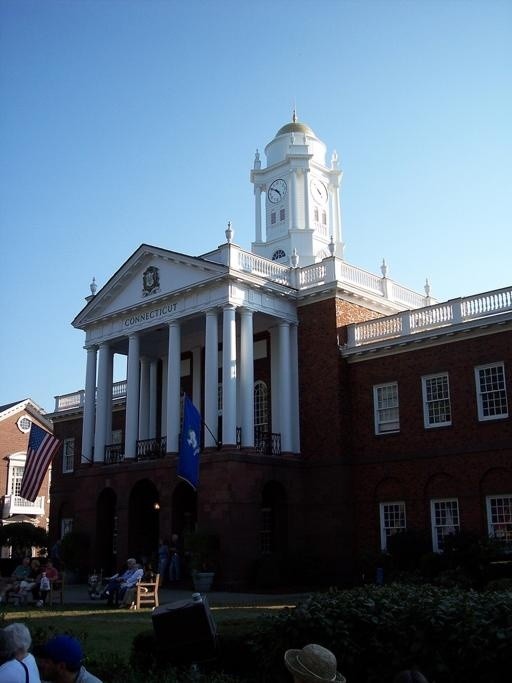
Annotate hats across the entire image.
[33,634,82,665]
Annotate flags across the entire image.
[177,395,203,491]
[18,421,63,501]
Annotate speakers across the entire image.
[150,595,217,664]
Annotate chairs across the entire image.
[134,574,162,610]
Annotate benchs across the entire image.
[3,571,66,606]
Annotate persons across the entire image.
[0,556,156,611]
[157,534,180,603]
[40,635,103,682]
[1,622,41,683]
[51,540,64,560]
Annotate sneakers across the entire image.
[0,595,45,609]
